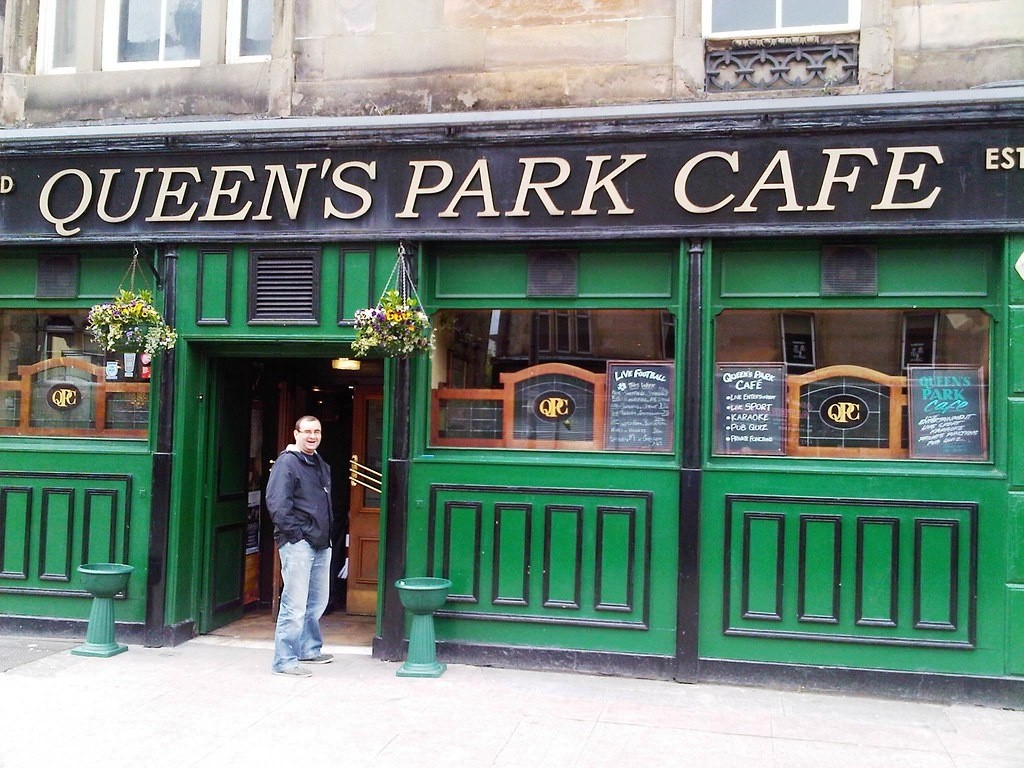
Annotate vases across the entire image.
[393,577,452,679]
[101,323,149,352]
[72,564,134,657]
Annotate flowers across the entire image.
[351,291,436,357]
[86,286,177,354]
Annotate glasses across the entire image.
[298,430,321,437]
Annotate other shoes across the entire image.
[297,654,333,664]
[271,665,312,678]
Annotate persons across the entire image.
[265,416,333,677]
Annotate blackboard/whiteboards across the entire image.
[906,363,986,459]
[603,357,673,453]
[713,359,787,464]
